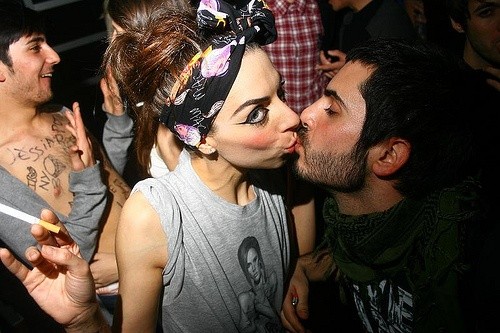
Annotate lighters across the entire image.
[292,292,299,307]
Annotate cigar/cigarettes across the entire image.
[0,203,60,234]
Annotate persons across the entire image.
[107,0,194,190]
[0,6,301,333]
[260,0,329,258]
[397,0,500,192]
[315,0,417,78]
[280,42,500,333]
[0,18,134,327]
